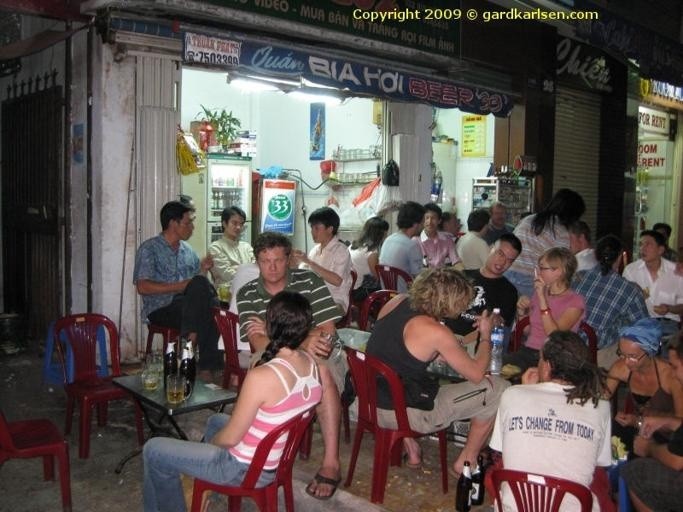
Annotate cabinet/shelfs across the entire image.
[471,177,532,227]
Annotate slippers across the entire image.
[305,472,341,500]
[403,451,422,468]
[447,462,463,479]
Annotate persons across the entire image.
[133,188,682,512]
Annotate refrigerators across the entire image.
[180,151,252,264]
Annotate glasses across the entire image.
[616,349,645,363]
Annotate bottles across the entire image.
[421,253,429,268]
[139,337,196,404]
[210,169,244,233]
[454,454,485,512]
[486,308,504,376]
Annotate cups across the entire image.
[215,283,232,302]
[315,330,342,362]
[334,149,370,183]
